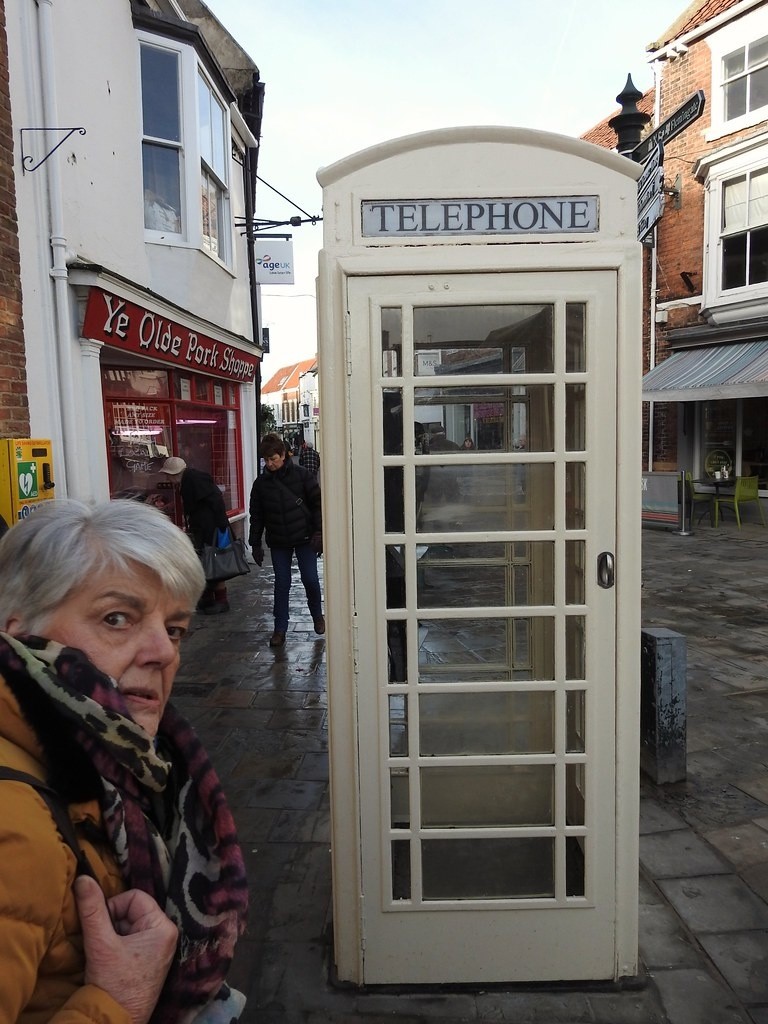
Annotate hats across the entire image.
[159,457,187,474]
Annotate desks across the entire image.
[690,478,742,529]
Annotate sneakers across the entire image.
[312,614,326,635]
[199,589,230,613]
[269,629,287,647]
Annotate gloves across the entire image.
[310,532,323,558]
[250,545,266,567]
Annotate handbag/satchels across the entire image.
[200,526,251,581]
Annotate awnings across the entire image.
[642,338,768,402]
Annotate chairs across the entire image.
[677,472,714,529]
[713,475,767,533]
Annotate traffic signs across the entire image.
[632,90,709,241]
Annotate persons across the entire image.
[248,433,324,646]
[279,406,538,529]
[161,457,234,614]
[1,497,248,1024]
